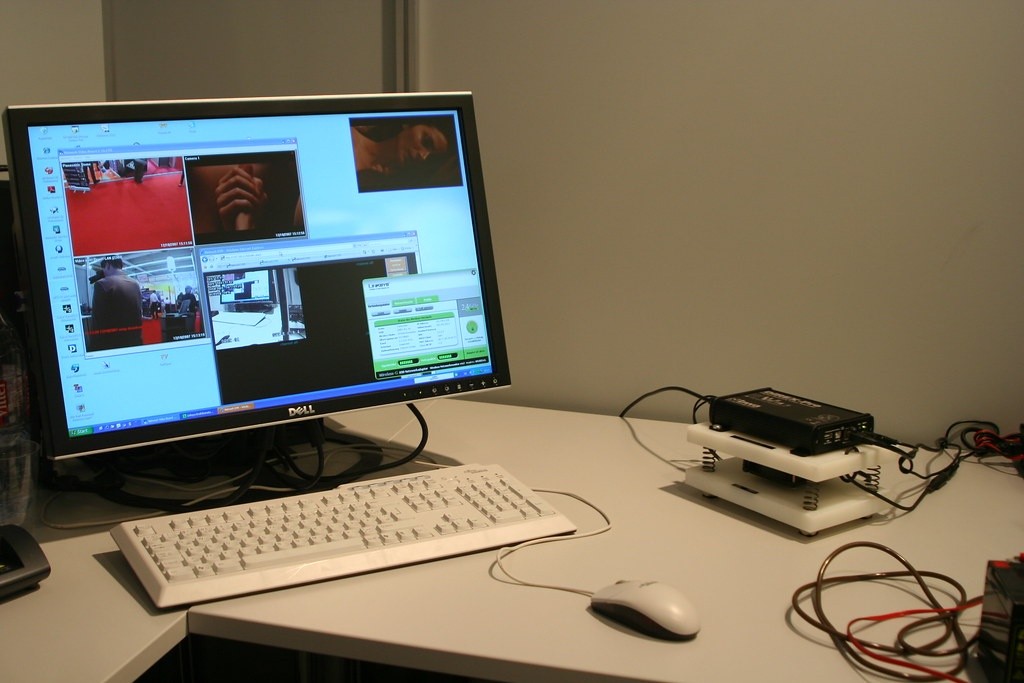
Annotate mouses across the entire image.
[590,580,702,640]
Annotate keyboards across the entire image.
[111,464,578,609]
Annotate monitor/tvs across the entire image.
[1,89,511,509]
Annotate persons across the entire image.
[350,118,453,195]
[187,164,271,234]
[145,285,198,320]
[92,255,143,352]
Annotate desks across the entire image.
[0,398,437,683]
[188,397,1023,683]
